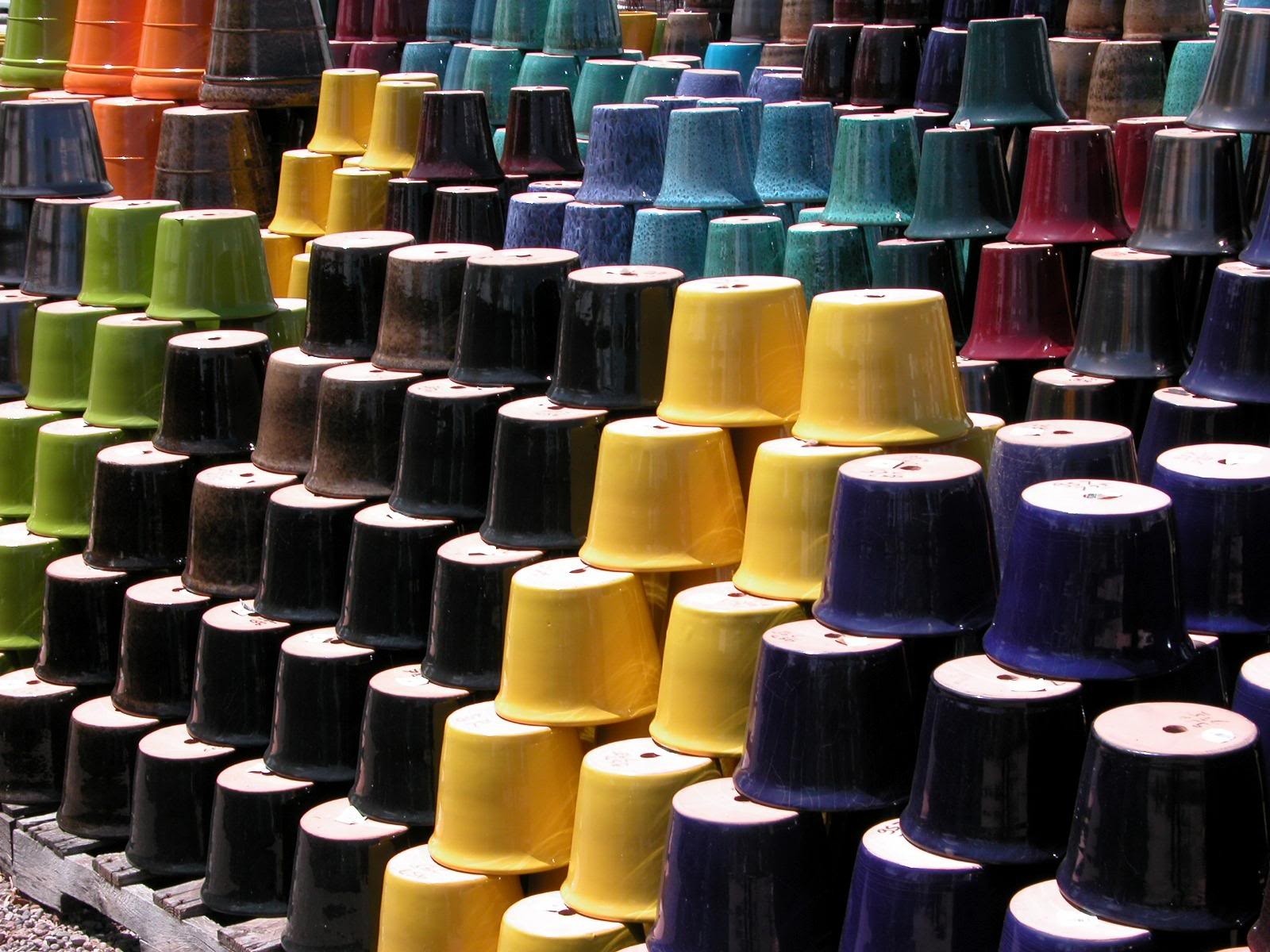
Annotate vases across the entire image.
[0,0,1270,952]
